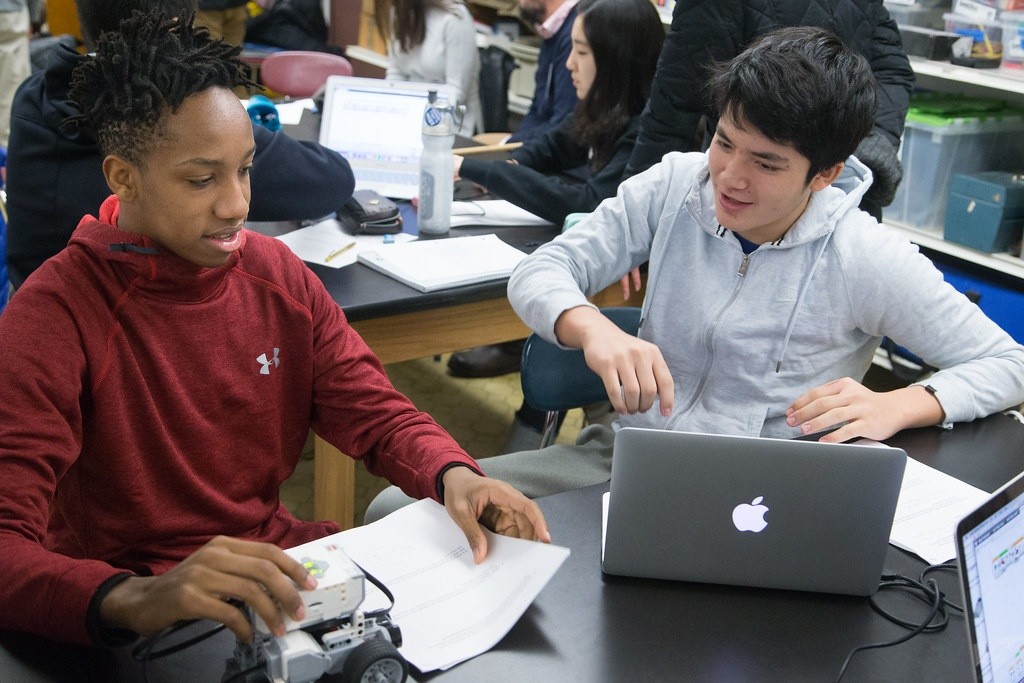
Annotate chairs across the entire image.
[520,307,642,450]
[260,51,353,104]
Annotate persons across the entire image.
[5,0,483,294]
[365,27,1024,526]
[446,0,917,377]
[0,6,551,650]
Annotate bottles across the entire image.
[418,101,455,234]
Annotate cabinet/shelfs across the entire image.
[873,56,1024,379]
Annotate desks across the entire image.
[244,103,648,539]
[0,381,1024,683]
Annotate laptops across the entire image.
[953,472,1024,683]
[319,76,457,201]
[601,426,908,597]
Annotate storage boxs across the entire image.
[882,0,1024,68]
[882,88,1024,236]
[944,170,1024,255]
